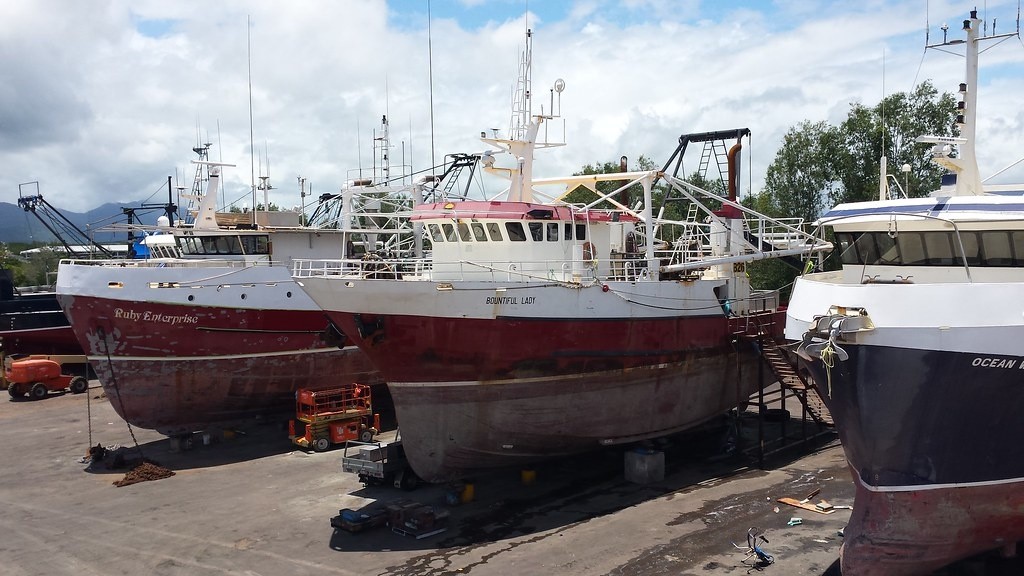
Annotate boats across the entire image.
[54,0,668,440]
[289,0,834,485]
[781,1,1024,576]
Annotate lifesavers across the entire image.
[580,242,595,261]
